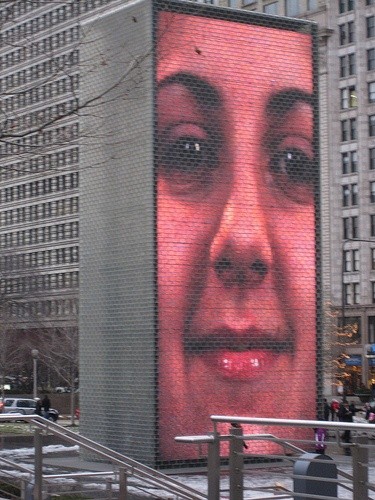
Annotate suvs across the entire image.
[0,397,59,423]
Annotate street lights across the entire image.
[31,348,38,400]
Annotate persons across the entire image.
[154,11,317,459]
[42,395,51,418]
[316,428,326,453]
[34,398,42,415]
[323,397,375,442]
